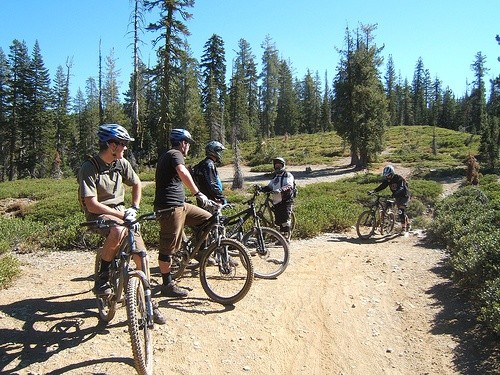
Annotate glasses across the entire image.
[112,141,128,147]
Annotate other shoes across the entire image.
[400,228,405,236]
[407,223,411,231]
[230,258,239,267]
[275,240,290,246]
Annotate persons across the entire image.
[154,129,215,296]
[257,158,294,244]
[80,124,166,324]
[368,167,411,235]
[191,142,238,266]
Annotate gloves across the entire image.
[194,192,208,208]
[127,216,141,233]
[124,206,138,219]
[274,189,281,195]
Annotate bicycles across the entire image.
[250,185,296,241]
[357,193,396,239]
[199,204,289,278]
[80,207,174,375]
[171,223,254,303]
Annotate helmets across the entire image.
[382,167,394,180]
[98,123,135,144]
[170,128,195,144]
[205,141,227,164]
[272,158,286,174]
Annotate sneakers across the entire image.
[161,283,188,296]
[138,302,165,324]
[95,279,112,295]
[198,252,216,266]
